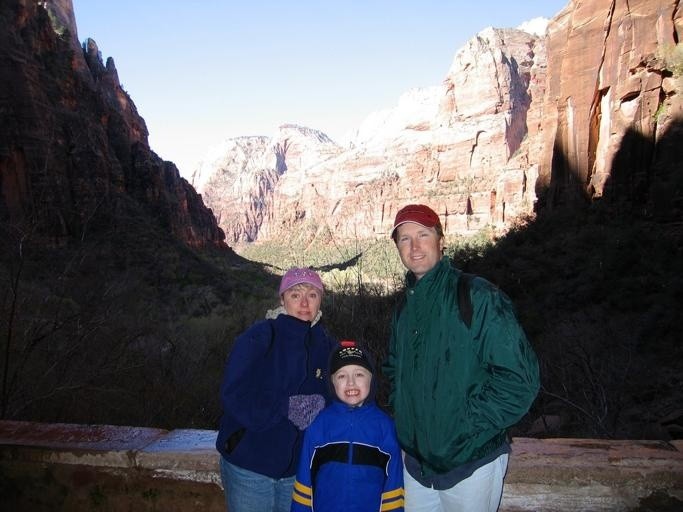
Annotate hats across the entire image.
[330,346,372,375]
[279,267,323,296]
[390,204,439,239]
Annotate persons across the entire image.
[215,266,336,511]
[288,342,402,511]
[380,204,543,512]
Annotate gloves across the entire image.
[288,394,325,432]
